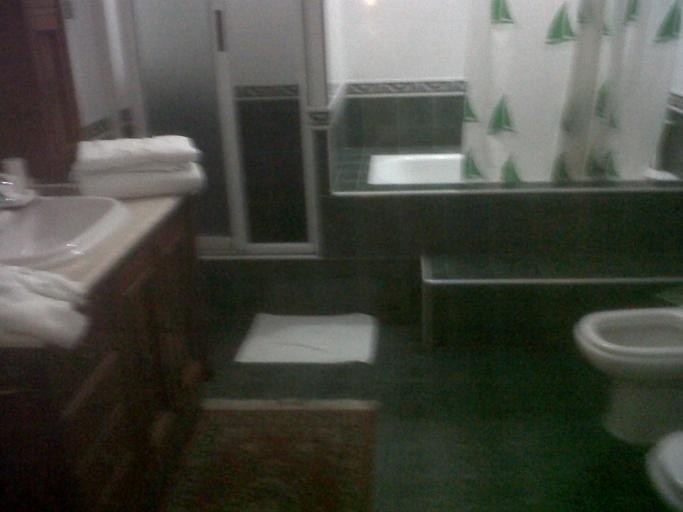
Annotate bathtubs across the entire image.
[363,152,683,188]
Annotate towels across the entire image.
[0,266,89,349]
[77,163,207,199]
[65,135,204,182]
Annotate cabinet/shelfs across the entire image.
[0,198,212,512]
[0,0,88,183]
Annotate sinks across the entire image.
[0,192,122,264]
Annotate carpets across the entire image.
[157,398,380,512]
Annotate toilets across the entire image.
[646,431,683,511]
[573,306,683,441]
